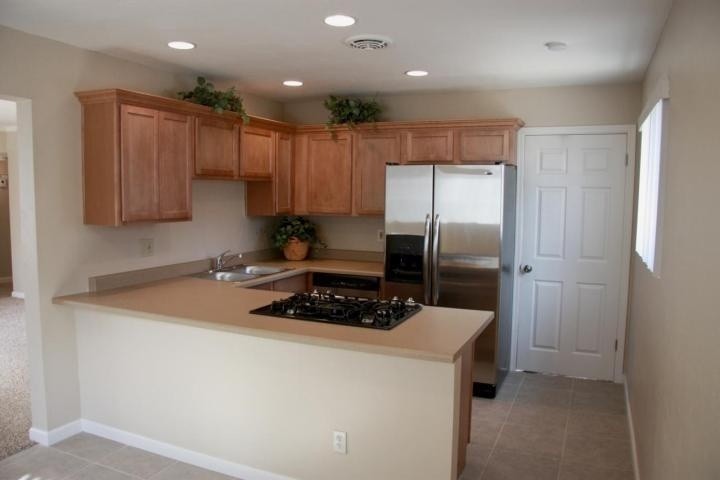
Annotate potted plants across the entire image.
[267,215,328,261]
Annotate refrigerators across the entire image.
[379,158,519,401]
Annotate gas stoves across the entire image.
[246,290,426,330]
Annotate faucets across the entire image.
[214,249,242,271]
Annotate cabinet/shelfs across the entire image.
[406,127,510,163]
[195,115,273,178]
[83,103,193,227]
[247,132,292,218]
[291,126,403,216]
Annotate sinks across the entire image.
[207,271,255,282]
[244,265,285,277]
